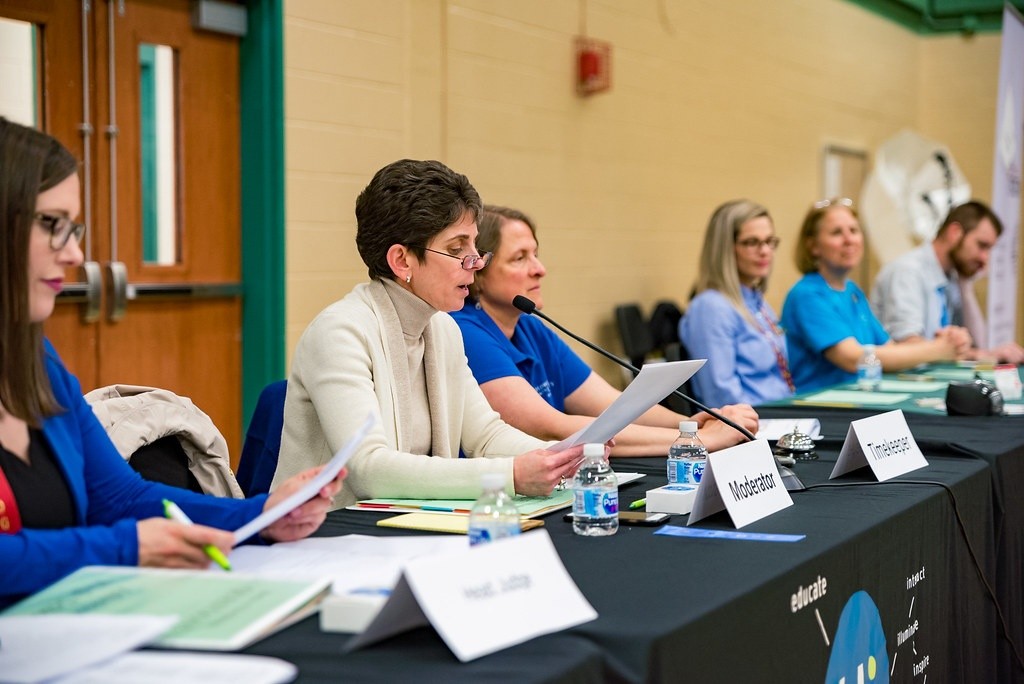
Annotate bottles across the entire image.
[469,472,520,547]
[573,443,619,536]
[857,344,882,391]
[667,422,708,484]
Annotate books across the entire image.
[1,565,336,652]
[377,508,543,535]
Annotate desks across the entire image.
[4,357,1024,684]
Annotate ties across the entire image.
[939,286,948,326]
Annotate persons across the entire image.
[780,195,972,394]
[0,116,349,600]
[867,200,1024,365]
[677,196,796,412]
[448,204,759,458]
[270,157,617,513]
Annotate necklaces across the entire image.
[755,305,795,393]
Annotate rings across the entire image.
[562,475,566,479]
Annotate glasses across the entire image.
[33,211,85,250]
[735,237,780,251]
[815,198,856,214]
[424,245,491,270]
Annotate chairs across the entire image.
[235,380,286,499]
[83,385,245,504]
[617,302,698,418]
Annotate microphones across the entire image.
[512,295,808,488]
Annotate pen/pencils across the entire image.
[162,498,232,571]
[629,498,647,509]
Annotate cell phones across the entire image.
[563,512,671,527]
